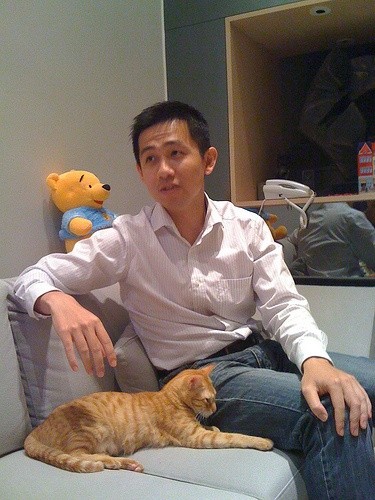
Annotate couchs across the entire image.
[0,276,375,500]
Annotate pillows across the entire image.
[112,320,161,393]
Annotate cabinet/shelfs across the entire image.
[225,0,375,358]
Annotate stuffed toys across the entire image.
[246,207,287,241]
[46,169,120,254]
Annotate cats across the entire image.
[23,361,274,473]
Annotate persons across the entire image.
[12,100,375,500]
[289,201,375,278]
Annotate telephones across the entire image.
[263,179,316,199]
